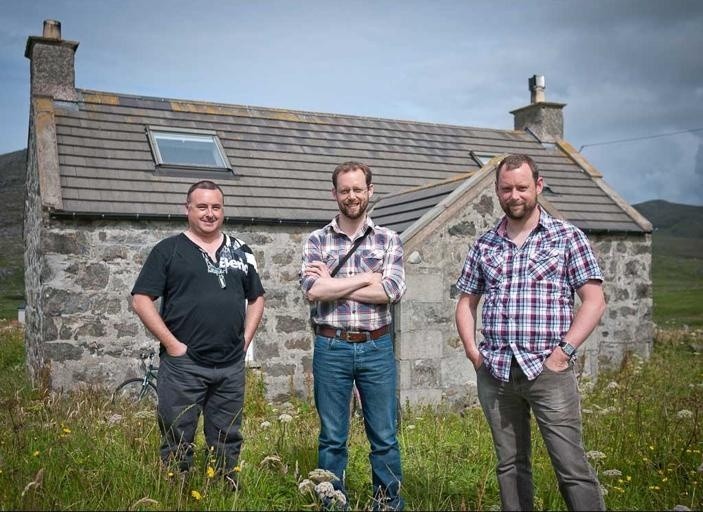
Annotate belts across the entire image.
[314,324,391,343]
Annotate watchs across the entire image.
[558,341,577,357]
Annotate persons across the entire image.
[456,153,607,512]
[132,181,266,490]
[303,161,405,512]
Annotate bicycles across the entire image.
[111,344,263,420]
